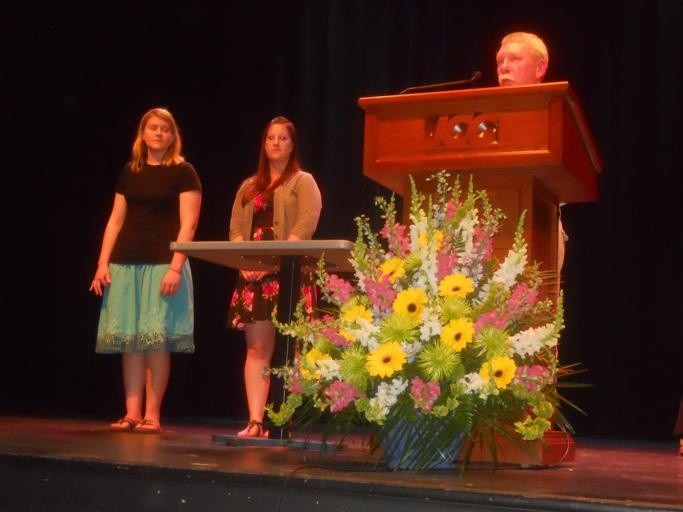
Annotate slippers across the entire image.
[111,418,160,433]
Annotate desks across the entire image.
[166,234,369,456]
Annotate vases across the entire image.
[369,402,481,472]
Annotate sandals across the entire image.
[247,421,263,437]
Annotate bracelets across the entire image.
[168,267,180,274]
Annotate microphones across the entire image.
[399,71,483,94]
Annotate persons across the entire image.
[225,113,322,438]
[88,107,202,432]
[496,31,566,293]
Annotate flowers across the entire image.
[260,168,593,474]
